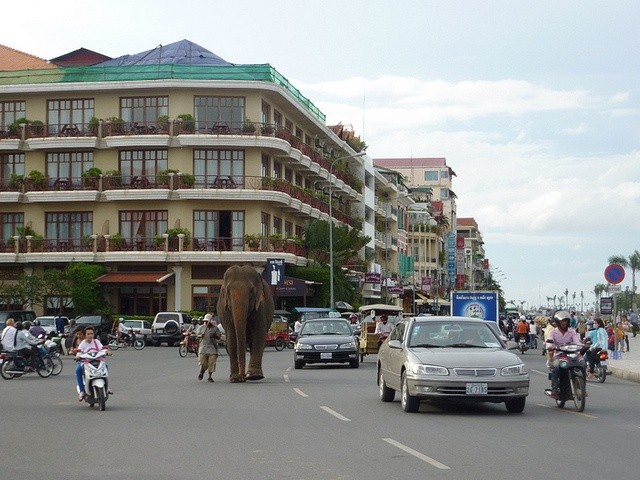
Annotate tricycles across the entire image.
[360,317,368,363]
[261,316,289,352]
[176,319,206,357]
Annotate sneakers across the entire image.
[208,378,214,382]
[199,374,203,380]
[551,389,560,399]
[78,392,85,401]
[107,388,113,394]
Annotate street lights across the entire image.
[329,151,366,309]
[385,195,409,302]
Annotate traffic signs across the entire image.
[602,260,627,284]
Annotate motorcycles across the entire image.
[107,326,145,350]
[518,333,530,355]
[587,348,617,388]
[507,329,513,340]
[72,329,112,410]
[376,332,390,351]
[0,343,63,380]
[542,337,592,412]
[45,343,63,375]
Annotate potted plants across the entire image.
[149,236,165,250]
[27,170,47,191]
[179,113,194,135]
[279,125,367,195]
[105,170,122,188]
[242,119,255,134]
[6,236,15,252]
[156,116,169,134]
[157,170,169,189]
[113,233,125,250]
[275,178,351,225]
[81,234,92,251]
[29,120,45,138]
[7,116,23,138]
[245,233,263,251]
[262,176,272,190]
[82,168,103,190]
[263,125,274,136]
[269,233,284,252]
[286,236,308,256]
[180,174,196,189]
[10,175,20,190]
[165,228,191,249]
[107,116,125,136]
[85,116,100,137]
[16,226,42,253]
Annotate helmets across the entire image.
[349,314,357,319]
[585,320,594,325]
[554,311,571,321]
[22,321,31,328]
[33,319,40,325]
[520,315,526,321]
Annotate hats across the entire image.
[119,318,124,322]
[192,318,200,321]
[379,313,388,318]
[14,321,22,329]
[201,313,215,324]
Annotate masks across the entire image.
[593,324,600,328]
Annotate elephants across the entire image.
[215,264,276,383]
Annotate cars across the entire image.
[2,310,36,328]
[35,316,69,337]
[291,317,361,370]
[149,312,185,346]
[82,342,88,345]
[117,319,150,344]
[376,316,530,414]
[69,315,109,337]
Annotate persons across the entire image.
[294,317,303,334]
[582,318,609,373]
[545,309,590,399]
[69,326,114,402]
[529,321,537,349]
[197,314,221,383]
[542,318,555,367]
[117,317,133,348]
[622,315,632,352]
[499,315,515,334]
[605,320,612,329]
[0,317,47,370]
[515,315,530,344]
[570,311,577,329]
[349,313,363,341]
[375,315,395,352]
[61,318,77,338]
[186,317,200,346]
[615,322,624,352]
[629,310,638,337]
